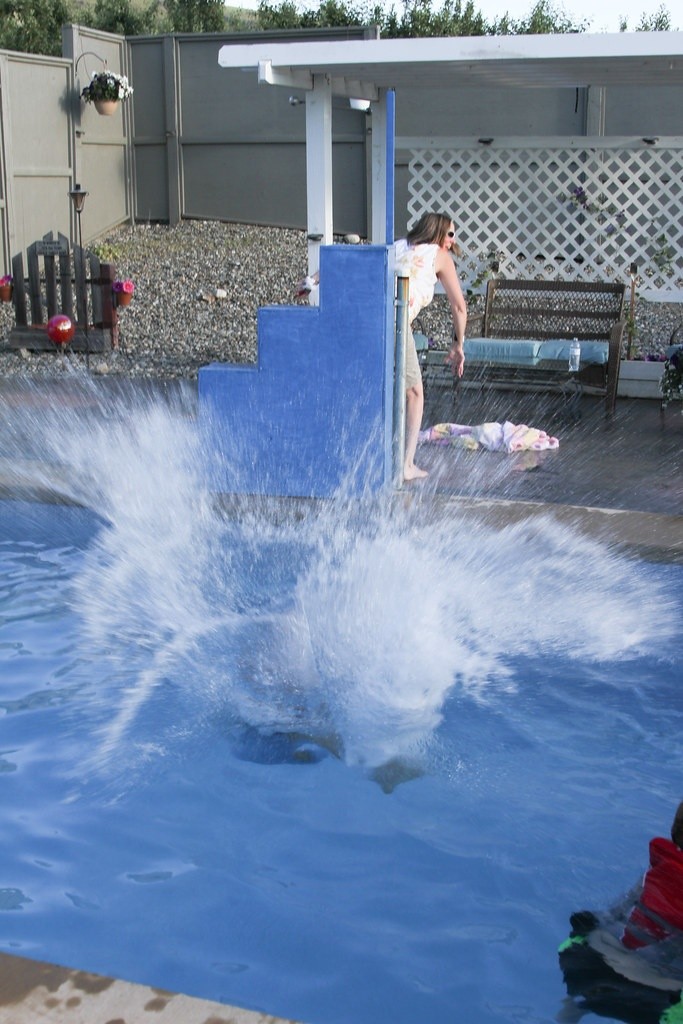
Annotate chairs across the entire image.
[661,322,683,410]
[412,318,428,395]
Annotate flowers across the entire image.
[113,280,134,294]
[624,308,668,362]
[0,274,13,287]
[79,69,135,105]
[658,352,683,409]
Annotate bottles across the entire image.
[569,338,581,371]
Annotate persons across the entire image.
[393,214,467,480]
[620,801,683,948]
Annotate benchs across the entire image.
[452,279,625,420]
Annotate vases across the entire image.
[617,360,683,399]
[0,286,13,301]
[118,292,131,305]
[94,99,119,115]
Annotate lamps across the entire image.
[70,184,87,212]
[643,137,658,145]
[479,137,493,144]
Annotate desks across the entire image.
[421,358,598,425]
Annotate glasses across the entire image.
[447,232,454,237]
